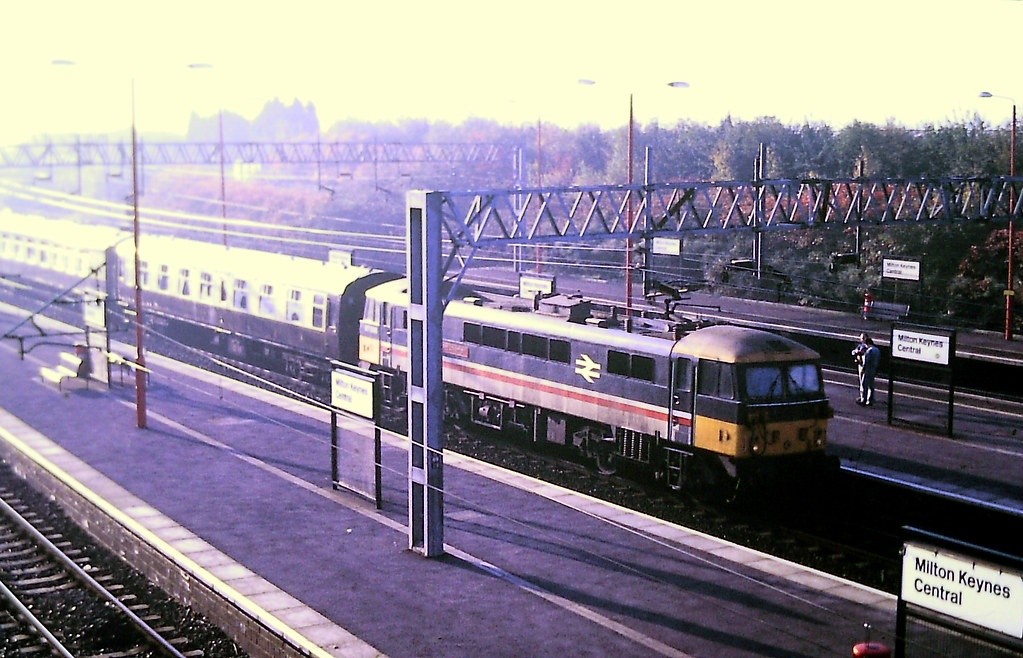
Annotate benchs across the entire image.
[39,350,83,392]
[858,300,911,326]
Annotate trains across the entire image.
[0,206,834,520]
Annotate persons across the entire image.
[850,332,869,401]
[854,337,879,405]
